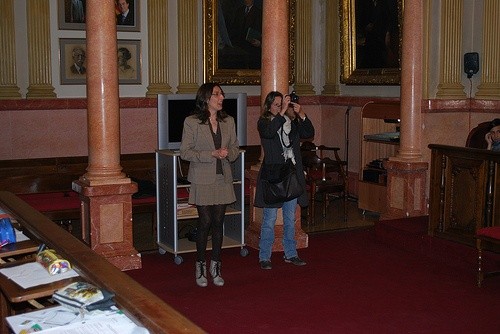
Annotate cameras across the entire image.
[284,92,300,108]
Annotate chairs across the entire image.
[475,224,500,288]
[311,145,347,226]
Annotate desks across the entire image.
[0,190,216,334]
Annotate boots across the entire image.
[209,258,225,286]
[195,260,208,287]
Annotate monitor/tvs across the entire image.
[157,94,247,152]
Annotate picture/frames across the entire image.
[338,0,405,86]
[58,0,86,30]
[202,0,296,86]
[117,39,142,85]
[60,38,87,85]
[115,0,141,32]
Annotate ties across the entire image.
[121,15,125,23]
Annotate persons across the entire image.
[255,91,314,268]
[180,82,240,287]
[485,118,500,149]
[116,0,134,26]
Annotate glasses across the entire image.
[211,92,224,98]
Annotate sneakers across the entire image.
[259,259,273,270]
[284,256,307,266]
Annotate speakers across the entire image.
[463,52,480,73]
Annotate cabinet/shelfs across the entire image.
[357,101,402,222]
[156,151,249,263]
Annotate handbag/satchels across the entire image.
[261,159,304,205]
[52,281,116,317]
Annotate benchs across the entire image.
[0,145,311,252]
